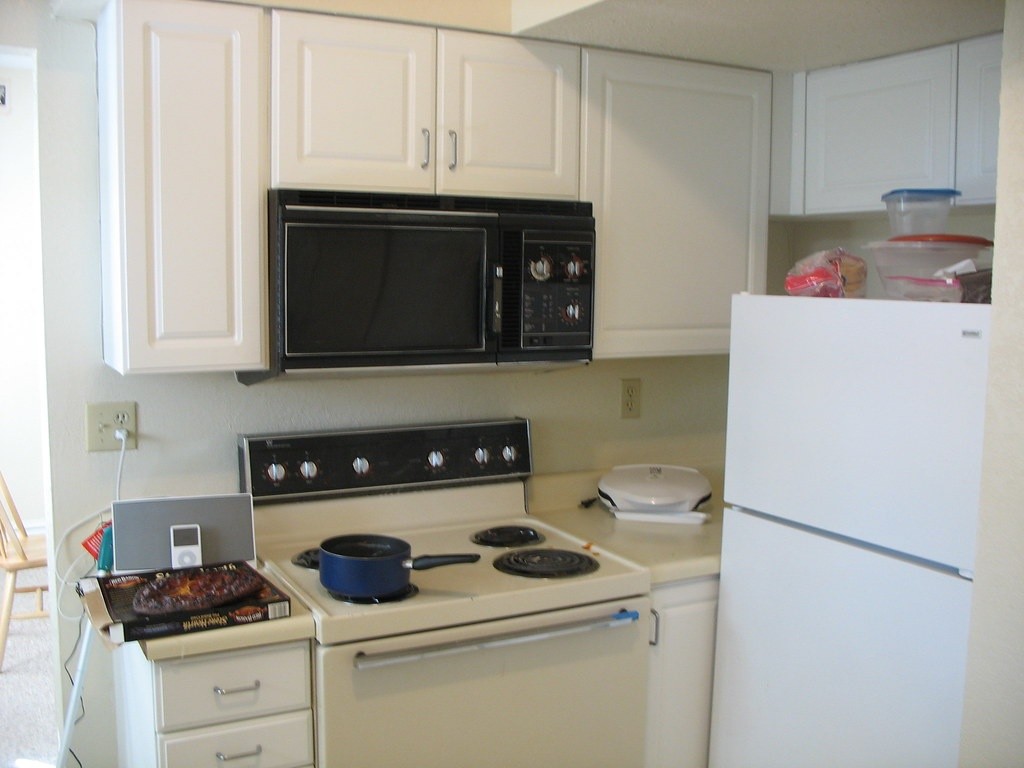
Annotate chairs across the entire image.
[0,470,49,673]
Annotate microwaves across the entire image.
[267,189,595,363]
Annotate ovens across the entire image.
[315,597,653,768]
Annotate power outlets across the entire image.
[85,399,138,453]
[620,377,641,420]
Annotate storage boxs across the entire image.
[861,235,994,289]
[881,188,962,236]
[78,558,291,651]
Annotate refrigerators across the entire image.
[709,291,991,768]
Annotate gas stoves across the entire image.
[239,415,650,644]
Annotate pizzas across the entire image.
[132,569,261,614]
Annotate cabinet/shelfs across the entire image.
[152,640,314,768]
[645,579,719,768]
[805,32,1003,217]
[97,0,271,376]
[581,47,773,361]
[271,9,580,201]
